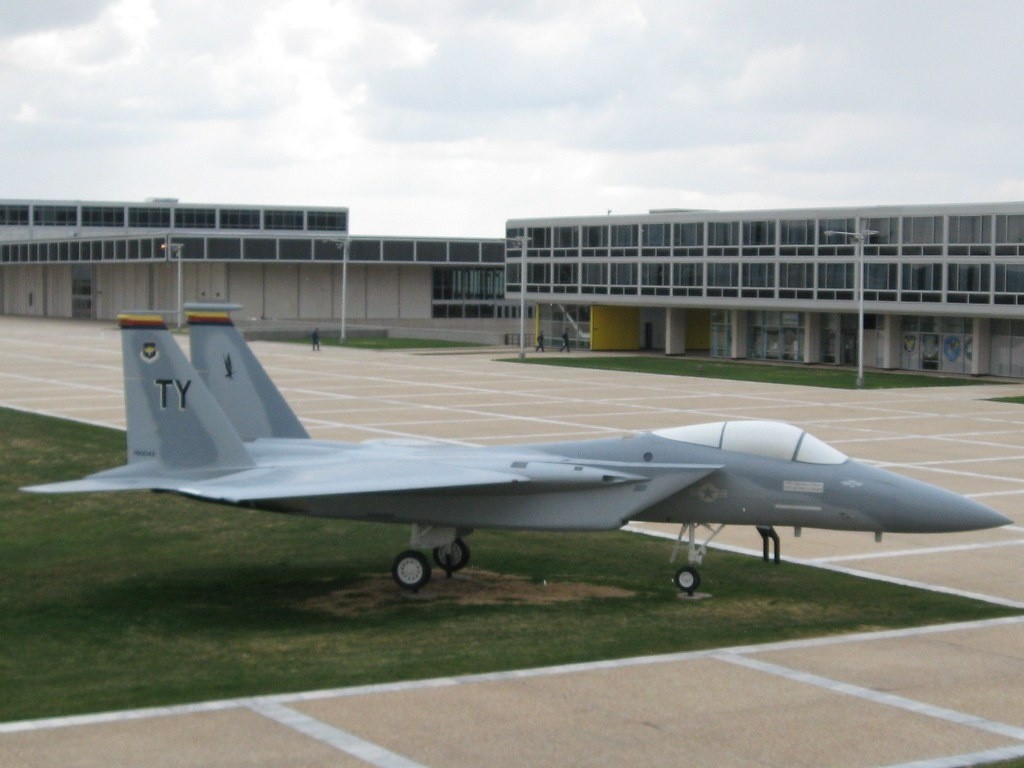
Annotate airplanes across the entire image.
[18,311,1013,590]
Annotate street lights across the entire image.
[161,244,182,327]
[500,237,527,358]
[322,238,348,343]
[825,231,865,386]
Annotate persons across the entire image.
[311,328,320,350]
[560,328,571,352]
[535,330,545,351]
[756,526,780,562]
